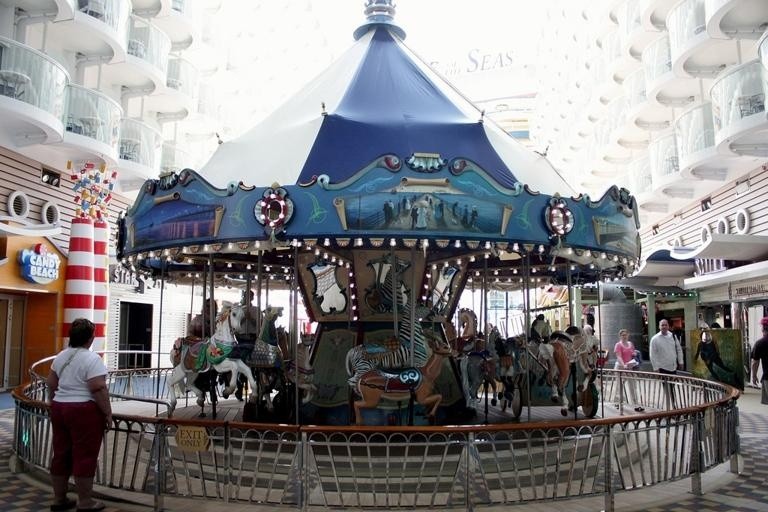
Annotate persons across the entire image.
[188,299,218,341]
[694,328,734,382]
[613,330,644,411]
[751,317,768,405]
[531,314,544,342]
[647,319,684,408]
[228,290,261,359]
[669,321,674,333]
[47,319,113,511]
[582,325,598,367]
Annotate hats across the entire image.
[759,317,768,326]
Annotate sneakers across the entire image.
[634,407,645,412]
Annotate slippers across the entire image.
[78,499,104,511]
[50,496,77,511]
